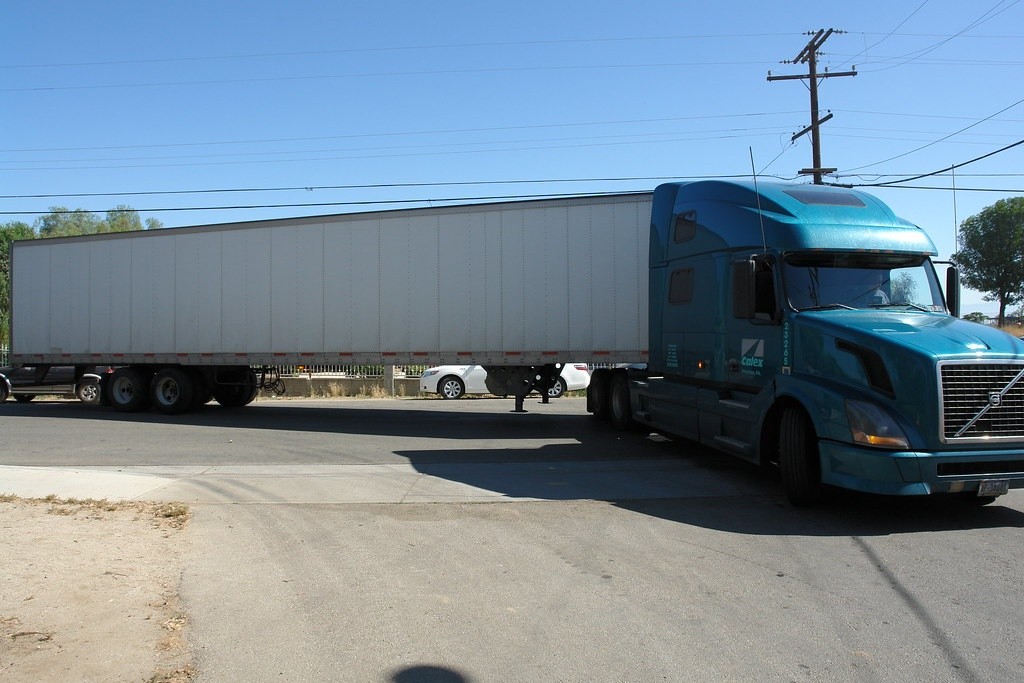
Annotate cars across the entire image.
[420,363,591,398]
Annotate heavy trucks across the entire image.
[7,181,1023,517]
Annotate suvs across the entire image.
[0,364,121,406]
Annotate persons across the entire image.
[865,274,889,305]
